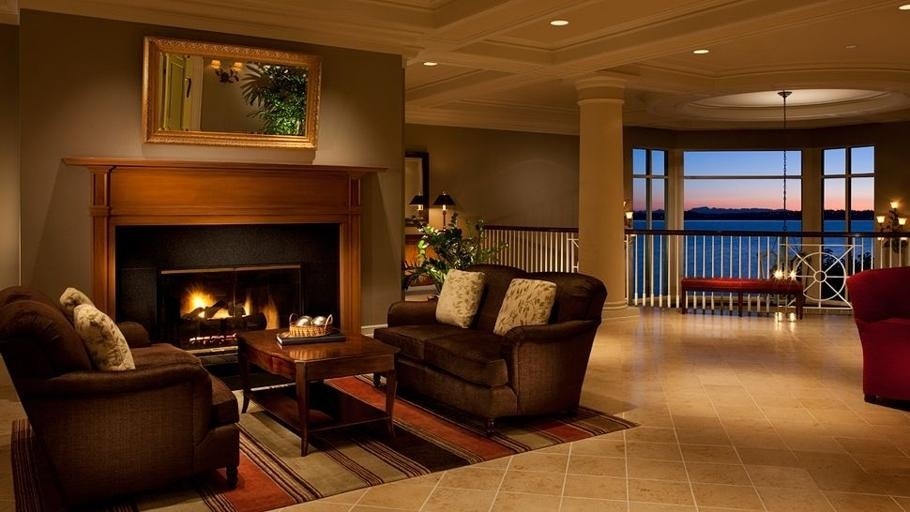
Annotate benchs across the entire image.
[680,277,804,319]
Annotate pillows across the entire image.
[72,303,136,370]
[58,287,95,326]
[493,278,557,336]
[435,268,489,330]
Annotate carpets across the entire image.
[11,373,640,512]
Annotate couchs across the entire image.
[0,284,241,509]
[371,263,607,437]
[847,266,910,405]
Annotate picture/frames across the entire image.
[141,35,323,151]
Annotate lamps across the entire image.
[433,190,456,234]
[410,193,422,220]
[876,200,908,254]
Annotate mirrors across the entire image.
[405,150,429,228]
